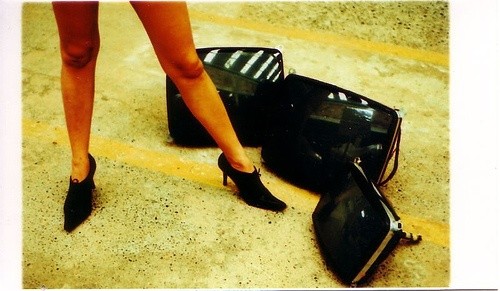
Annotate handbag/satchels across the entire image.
[312,157,423,287]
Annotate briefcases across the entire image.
[166,47,284,148]
[261,74,403,195]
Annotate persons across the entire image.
[50,1,287,233]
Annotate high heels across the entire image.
[64,153,97,232]
[218,152,286,211]
